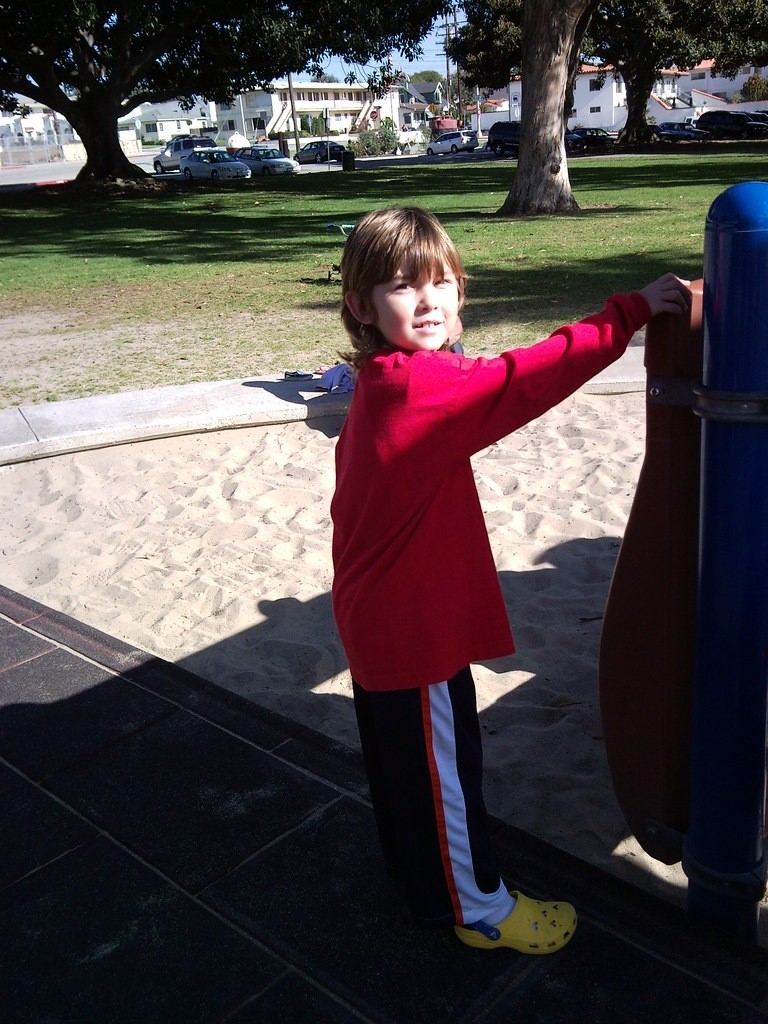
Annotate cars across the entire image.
[571,127,615,148]
[644,124,680,144]
[564,126,591,157]
[293,140,345,165]
[426,129,479,156]
[233,146,301,176]
[659,121,711,141]
[179,149,252,183]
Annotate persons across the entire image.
[331,205,693,954]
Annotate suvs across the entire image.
[152,133,218,174]
[684,110,768,140]
[487,121,522,159]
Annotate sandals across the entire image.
[454,890,577,955]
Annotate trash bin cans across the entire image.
[341,150,355,171]
